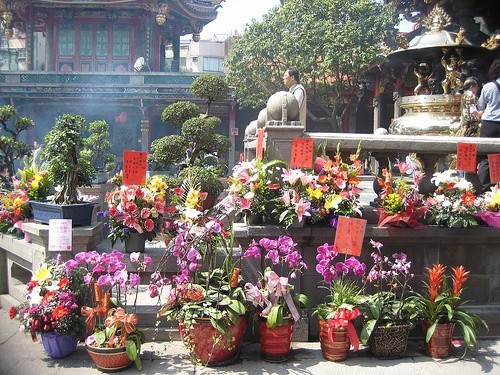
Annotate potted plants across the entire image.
[26,114,111,226]
[176,165,221,211]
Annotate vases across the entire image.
[372,322,414,357]
[422,322,456,358]
[178,312,245,365]
[42,333,76,357]
[319,318,351,362]
[290,214,307,229]
[258,311,295,359]
[260,205,277,225]
[123,230,147,253]
[247,211,262,225]
[85,332,133,370]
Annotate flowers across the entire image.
[99,161,500,249]
[0,151,53,232]
[5,232,488,370]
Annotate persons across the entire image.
[478,59,500,138]
[32,141,49,168]
[456,79,483,137]
[283,68,307,132]
